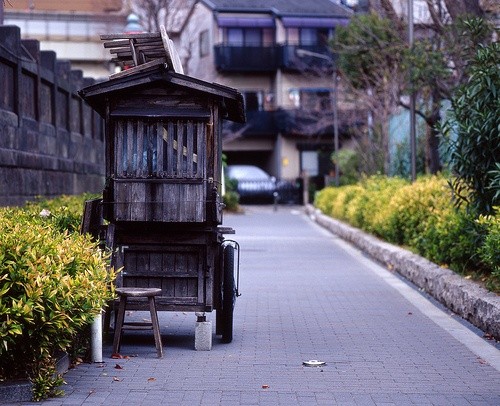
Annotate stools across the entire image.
[114,287,164,360]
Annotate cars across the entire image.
[223,165,278,205]
